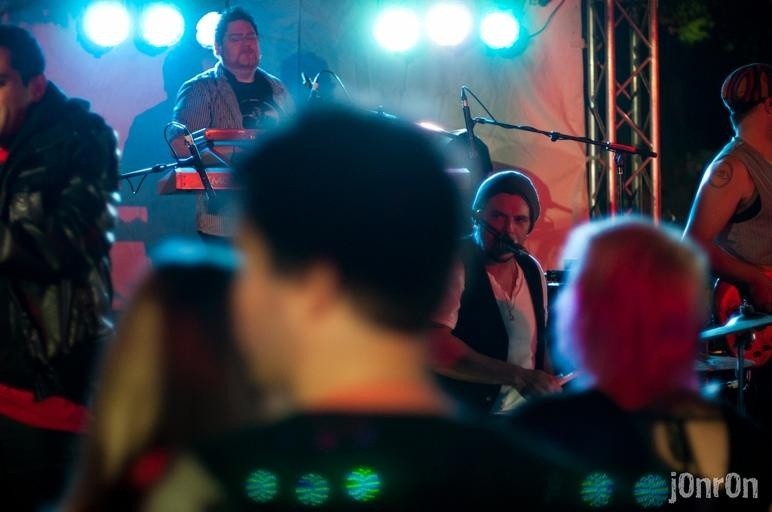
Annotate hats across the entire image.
[719,59,772,112]
[471,169,542,237]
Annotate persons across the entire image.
[2,5,772,511]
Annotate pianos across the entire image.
[156,129,252,195]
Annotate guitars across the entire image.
[709,263,772,366]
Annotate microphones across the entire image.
[184,131,217,202]
[459,90,480,161]
[476,215,519,256]
[305,79,319,104]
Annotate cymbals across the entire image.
[701,315,771,338]
[695,351,756,374]
